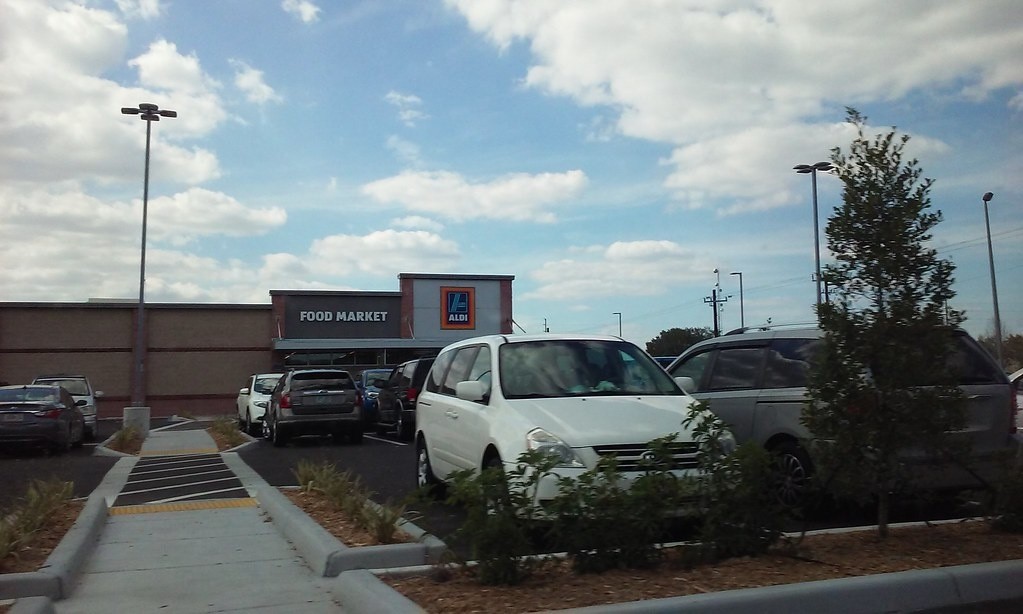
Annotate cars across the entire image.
[354,369,394,417]
[1005,367,1023,426]
[0,384,89,459]
[414,335,744,533]
[373,356,436,442]
[235,374,286,436]
[31,375,104,443]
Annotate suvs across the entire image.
[261,368,368,448]
[663,321,1019,521]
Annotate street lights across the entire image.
[791,159,836,325]
[731,272,744,329]
[120,104,178,407]
[983,191,1014,374]
[612,312,622,337]
